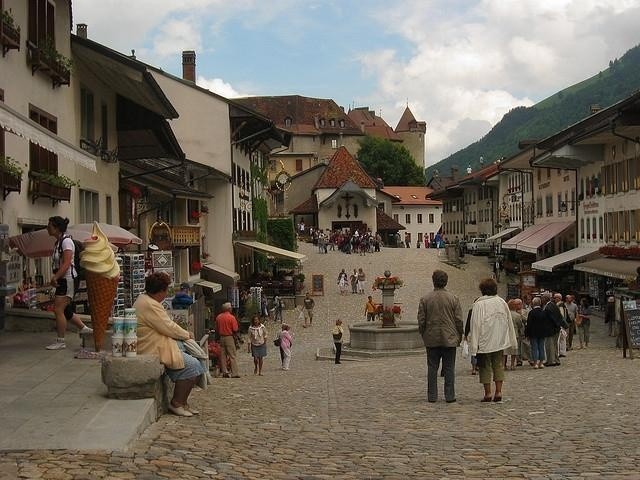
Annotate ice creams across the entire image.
[79,220,120,353]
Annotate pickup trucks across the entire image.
[465,237,490,256]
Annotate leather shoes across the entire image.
[481,395,492,401]
[494,395,502,402]
[516,361,560,368]
[223,373,229,377]
[167,404,193,416]
[184,405,199,414]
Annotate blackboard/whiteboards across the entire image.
[312,274,324,291]
[507,283,518,300]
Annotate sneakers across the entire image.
[78,327,94,334]
[45,342,65,349]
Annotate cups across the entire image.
[110,307,138,358]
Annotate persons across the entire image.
[300,292,315,328]
[131,271,208,417]
[337,267,366,294]
[248,315,269,377]
[240,288,284,325]
[394,232,460,248]
[332,319,344,365]
[417,270,464,403]
[46,216,93,350]
[216,301,241,378]
[364,296,377,321]
[279,324,293,371]
[462,256,591,401]
[298,219,382,256]
[604,296,615,337]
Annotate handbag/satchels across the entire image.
[275,339,281,346]
[333,333,341,340]
[462,340,469,360]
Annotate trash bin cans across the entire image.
[446,244,456,257]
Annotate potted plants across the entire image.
[1,7,21,45]
[0,156,28,189]
[36,34,73,81]
[34,168,80,201]
